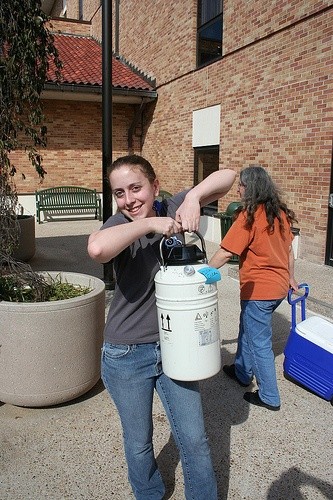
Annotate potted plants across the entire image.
[1,272,106,407]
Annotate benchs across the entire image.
[35,186,101,224]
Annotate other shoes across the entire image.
[223,364,251,387]
[243,390,281,411]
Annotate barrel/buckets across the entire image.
[154,230,221,381]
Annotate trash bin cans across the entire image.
[290,227,300,259]
[212,202,241,261]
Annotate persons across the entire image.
[87,155,236,500]
[209,167,298,411]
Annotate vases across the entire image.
[0,215,35,261]
